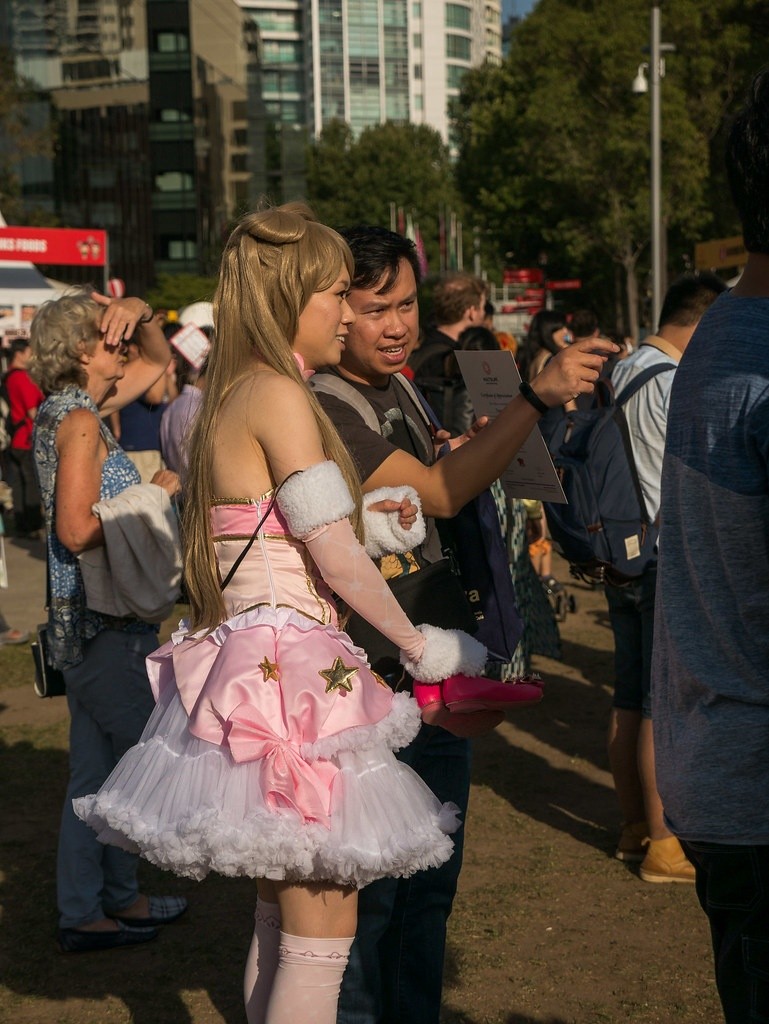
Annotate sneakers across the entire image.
[639,834,695,884]
[616,821,648,861]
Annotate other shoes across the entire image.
[114,893,187,927]
[443,671,545,713]
[0,627,29,644]
[413,679,505,737]
[56,916,159,955]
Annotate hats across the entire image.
[179,301,215,331]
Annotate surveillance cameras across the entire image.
[632,76,649,97]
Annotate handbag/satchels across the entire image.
[31,624,67,698]
[395,371,525,665]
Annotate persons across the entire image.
[308,223,620,1024]
[0,289,213,952]
[650,66,769,1024]
[66,203,488,1023]
[414,271,728,882]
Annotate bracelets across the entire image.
[518,382,553,416]
[139,306,155,323]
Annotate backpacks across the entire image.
[0,368,26,451]
[542,362,676,578]
